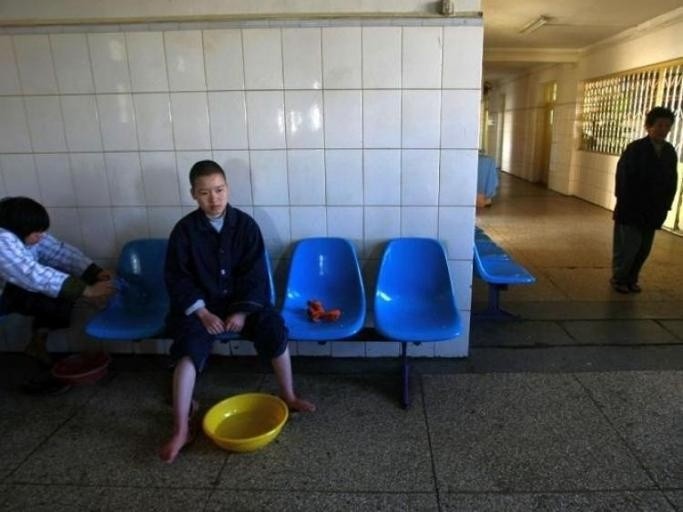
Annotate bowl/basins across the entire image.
[50,353,112,388]
[200,391,291,454]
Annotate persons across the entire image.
[157,160,315,463]
[610,106,677,294]
[1,194,115,367]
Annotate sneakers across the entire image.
[22,376,71,397]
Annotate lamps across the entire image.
[518,15,549,36]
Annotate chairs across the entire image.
[373,238,464,407]
[85,237,172,340]
[475,228,539,317]
[281,238,368,345]
[214,237,275,338]
